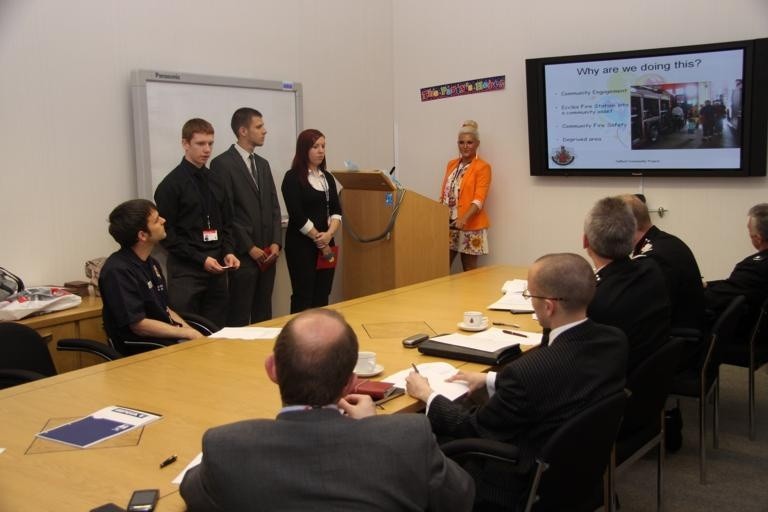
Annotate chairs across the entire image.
[103,302,221,357]
[0,321,124,390]
[610,337,683,512]
[672,295,748,484]
[439,387,632,512]
[719,292,768,441]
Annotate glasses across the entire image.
[523,288,565,301]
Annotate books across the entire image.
[430,326,544,354]
[380,361,471,403]
[487,278,535,315]
[35,402,164,447]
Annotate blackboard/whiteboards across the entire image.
[131,69,304,230]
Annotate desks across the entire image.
[0,265,543,512]
[329,169,450,301]
[16,289,108,376]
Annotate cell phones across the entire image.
[126,488,159,511]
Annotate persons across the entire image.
[281,129,342,314]
[178,311,478,512]
[98,199,206,354]
[583,197,672,376]
[406,252,628,510]
[153,118,239,329]
[443,120,492,272]
[703,204,764,348]
[211,108,281,326]
[619,192,707,347]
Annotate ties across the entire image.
[249,154,261,189]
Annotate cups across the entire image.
[354,350,376,372]
[463,311,488,328]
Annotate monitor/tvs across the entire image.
[524,39,768,177]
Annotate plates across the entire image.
[352,362,384,377]
[456,321,489,332]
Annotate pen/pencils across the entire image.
[502,329,527,338]
[493,322,522,329]
[411,363,420,374]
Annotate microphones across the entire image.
[389,166,396,175]
